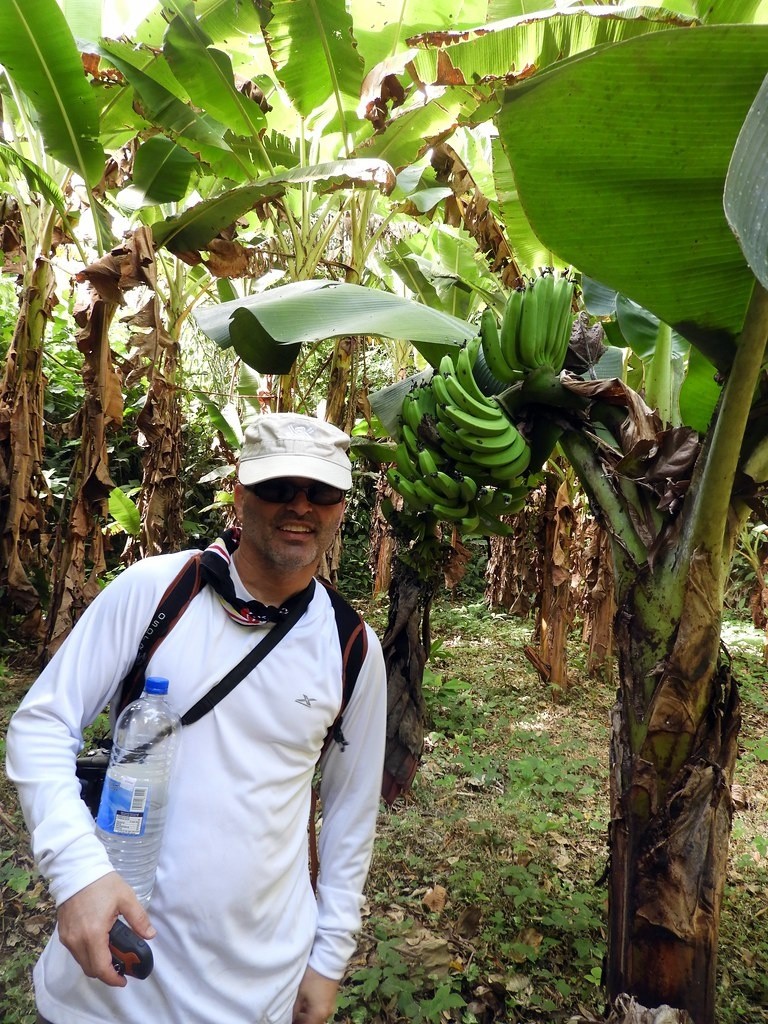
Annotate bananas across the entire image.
[381,267,581,541]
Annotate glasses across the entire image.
[245,479,344,506]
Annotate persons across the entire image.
[3,411,390,1024]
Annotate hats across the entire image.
[238,413,354,492]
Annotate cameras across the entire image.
[75,737,113,823]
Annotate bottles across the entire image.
[93,676,184,929]
